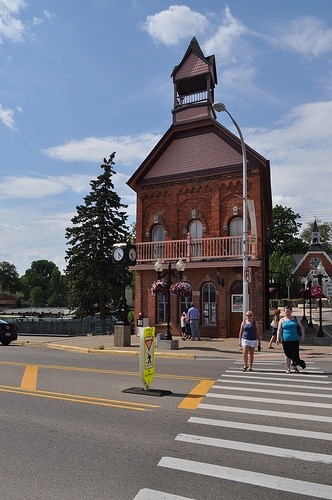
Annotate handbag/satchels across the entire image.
[294,316,302,335]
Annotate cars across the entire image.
[0,318,19,346]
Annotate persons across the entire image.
[239,310,261,373]
[267,309,282,349]
[275,304,306,373]
[181,313,188,340]
[182,308,191,339]
[187,302,201,341]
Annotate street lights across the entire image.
[154,258,185,340]
[314,260,325,337]
[212,100,251,318]
[301,276,308,325]
[308,273,314,327]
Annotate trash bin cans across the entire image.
[114,321,131,347]
[272,301,278,309]
[282,300,287,307]
[292,301,296,307]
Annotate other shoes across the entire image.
[248,369,252,372]
[286,369,291,373]
[293,367,300,373]
[242,366,247,372]
[269,346,275,349]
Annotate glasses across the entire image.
[246,315,253,317]
